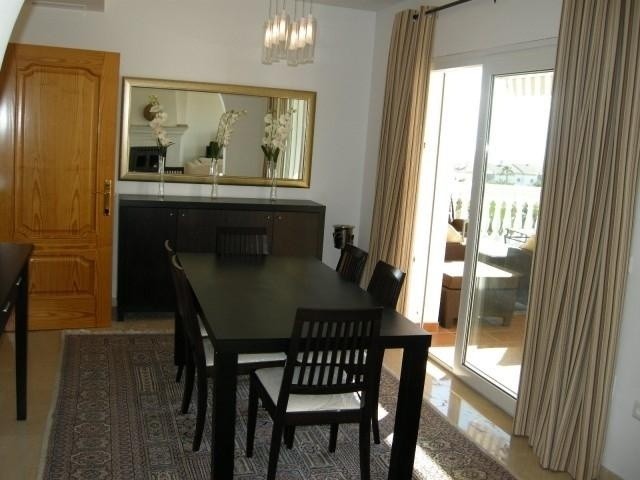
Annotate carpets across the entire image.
[39,327,515,480]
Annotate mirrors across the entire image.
[117,76,317,188]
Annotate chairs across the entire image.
[171,257,285,452]
[296,260,408,445]
[336,244,369,285]
[166,241,208,383]
[245,305,384,480]
[215,226,272,255]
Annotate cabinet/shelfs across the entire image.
[116,194,326,322]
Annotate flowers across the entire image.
[261,108,279,162]
[216,109,247,162]
[270,106,298,170]
[147,93,174,157]
[211,109,232,161]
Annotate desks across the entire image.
[0,243,36,421]
[177,257,431,479]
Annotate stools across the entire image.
[440,260,521,329]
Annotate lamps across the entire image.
[263,0,319,67]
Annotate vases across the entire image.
[265,162,271,178]
[209,164,213,175]
[159,157,165,196]
[270,164,277,200]
[212,164,218,198]
